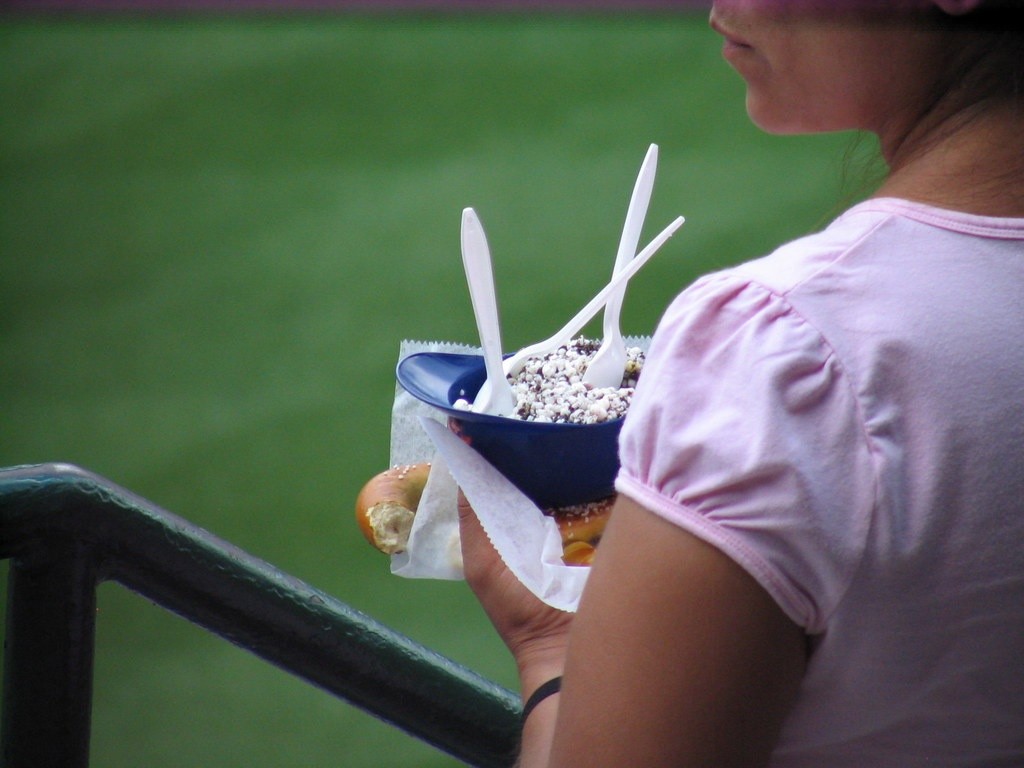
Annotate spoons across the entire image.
[503,214,687,376]
[458,204,517,419]
[587,141,661,392]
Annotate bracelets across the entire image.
[520,676,561,727]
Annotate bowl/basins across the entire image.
[396,351,638,508]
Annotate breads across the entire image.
[354,462,618,567]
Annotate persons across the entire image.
[450,0,1024,768]
